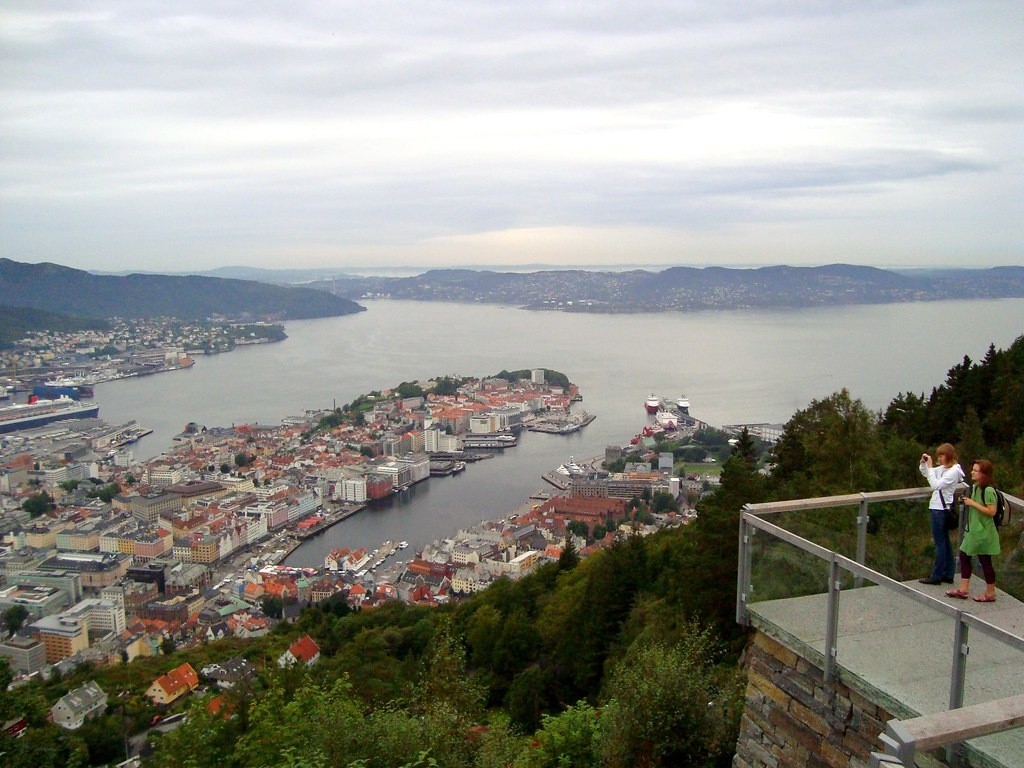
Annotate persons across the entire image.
[917,442,966,585]
[944,458,1002,602]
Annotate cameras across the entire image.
[956,494,966,505]
[923,455,928,461]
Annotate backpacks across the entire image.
[980,485,1011,529]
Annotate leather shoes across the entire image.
[938,576,954,584]
[919,578,941,585]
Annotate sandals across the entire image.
[946,588,968,599]
[972,592,996,602]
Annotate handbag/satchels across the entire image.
[946,507,959,531]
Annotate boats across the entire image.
[643,392,660,413]
[557,456,582,476]
[452,460,467,472]
[675,393,690,410]
[368,539,421,576]
